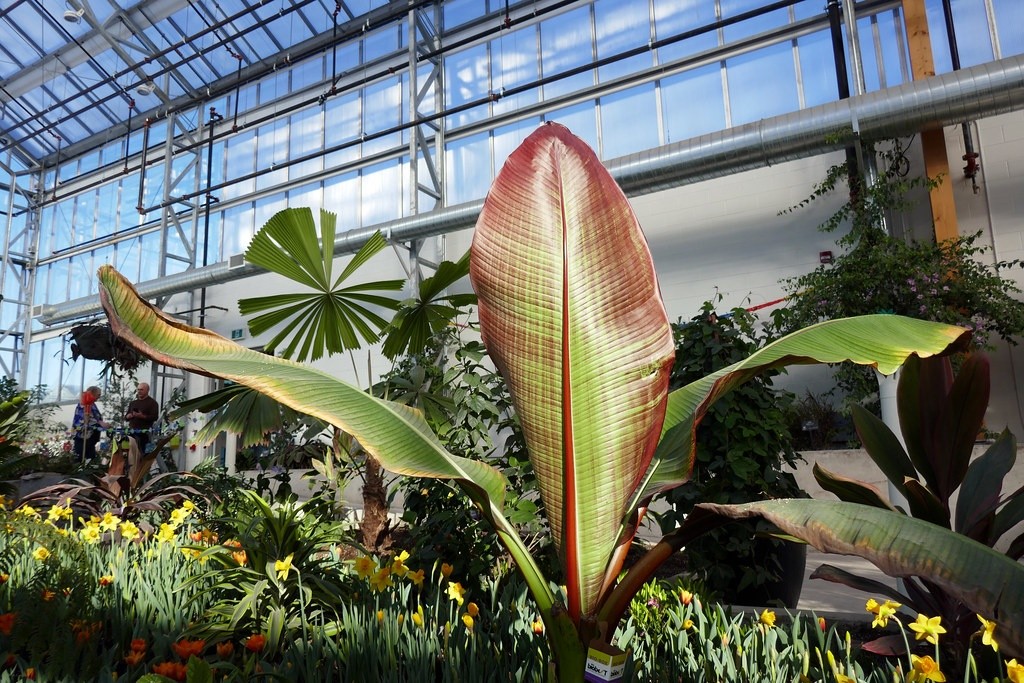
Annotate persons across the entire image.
[124,382,159,455]
[70,384,111,459]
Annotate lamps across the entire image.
[136,83,156,96]
[64,8,84,22]
